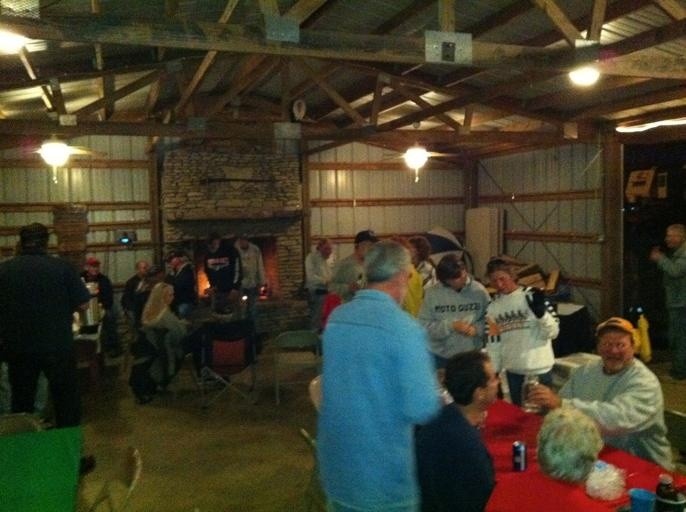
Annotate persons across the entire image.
[120,231,265,386]
[305,225,685,512]
[1,225,113,475]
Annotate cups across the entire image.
[629,487,657,512]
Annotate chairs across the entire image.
[0,413,41,433]
[298,424,327,512]
[665,409,686,462]
[89,446,143,512]
[271,331,320,403]
[307,373,322,410]
[192,316,258,411]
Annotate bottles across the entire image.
[518,372,544,414]
[657,474,680,502]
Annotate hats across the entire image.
[597,318,635,334]
[87,258,101,265]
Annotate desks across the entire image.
[71,316,102,401]
[0,424,86,512]
[556,299,591,357]
[473,397,686,512]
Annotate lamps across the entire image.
[38,135,70,182]
[403,142,429,185]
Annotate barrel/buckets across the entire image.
[77,279,99,325]
